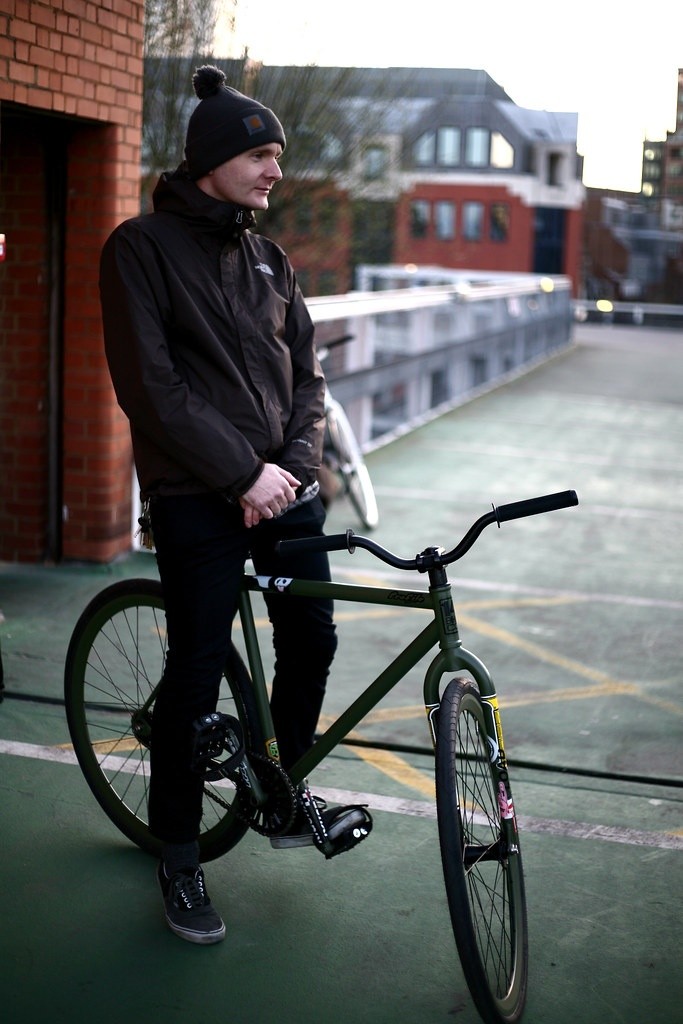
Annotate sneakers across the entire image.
[269,796,366,850]
[156,858,226,943]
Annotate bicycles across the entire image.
[63,488,580,1023]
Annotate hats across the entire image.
[184,64,287,182]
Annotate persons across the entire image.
[100,65,372,945]
